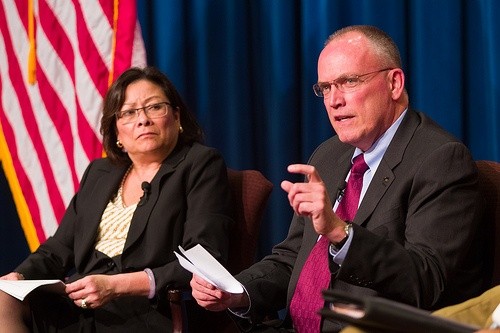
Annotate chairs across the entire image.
[167,167,274,333]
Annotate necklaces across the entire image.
[120,163,162,209]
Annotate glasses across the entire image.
[114,102,175,125]
[313,67,393,98]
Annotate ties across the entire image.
[287,152,369,333]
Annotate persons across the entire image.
[188,25,482,333]
[0,63,235,333]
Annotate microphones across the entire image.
[141,181,149,192]
[337,180,347,190]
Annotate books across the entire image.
[314,288,477,333]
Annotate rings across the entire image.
[81,299,88,309]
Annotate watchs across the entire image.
[331,219,353,250]
